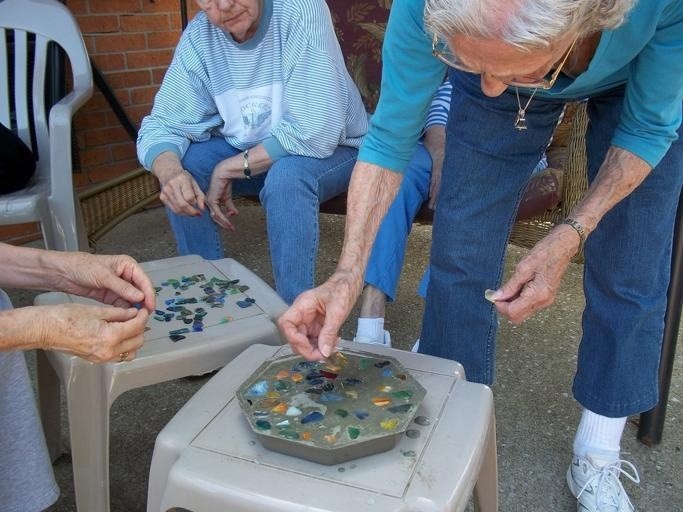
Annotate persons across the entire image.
[347,58,550,356]
[0,228,156,512]
[272,1,683,511]
[127,3,374,388]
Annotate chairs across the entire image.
[1,0,98,252]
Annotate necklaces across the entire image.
[511,87,544,134]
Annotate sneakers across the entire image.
[186,367,223,381]
[351,329,394,349]
[561,450,644,512]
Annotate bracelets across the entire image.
[558,216,587,264]
[241,144,251,183]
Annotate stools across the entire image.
[28,253,299,511]
[145,336,502,512]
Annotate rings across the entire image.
[117,352,128,363]
[209,211,215,218]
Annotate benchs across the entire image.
[239,1,682,450]
